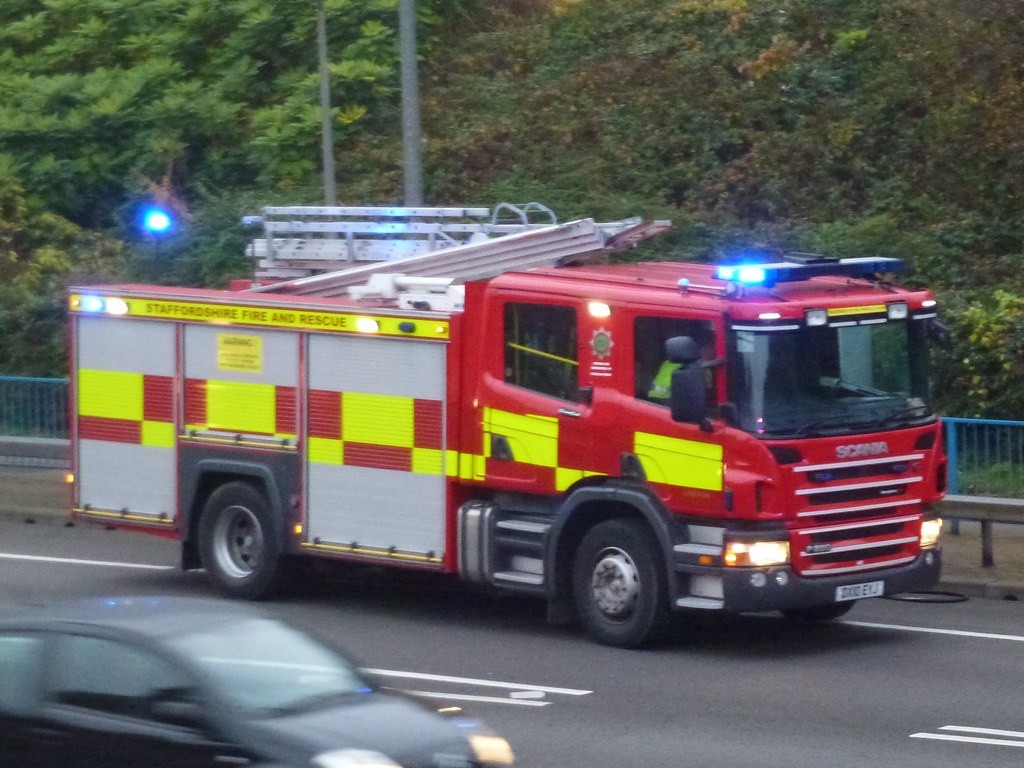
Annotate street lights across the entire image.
[143,208,171,282]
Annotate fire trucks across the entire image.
[65,205,951,650]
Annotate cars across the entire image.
[0,579,520,768]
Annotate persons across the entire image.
[647,328,715,407]
